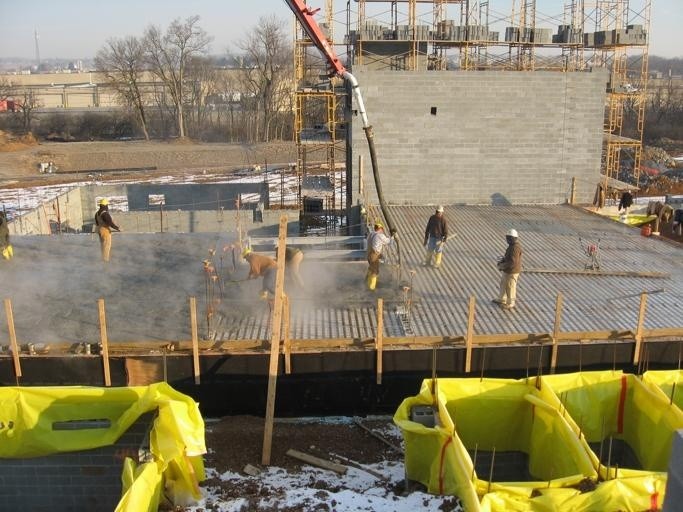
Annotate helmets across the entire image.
[100,199,108,206]
[241,249,250,258]
[436,206,444,212]
[506,229,518,238]
[374,224,382,230]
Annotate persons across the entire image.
[0,211,13,261]
[492,228,522,309]
[94,198,123,263]
[366,224,395,293]
[422,205,448,269]
[274,246,304,287]
[242,247,276,294]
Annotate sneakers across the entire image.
[503,304,513,308]
[493,299,506,304]
